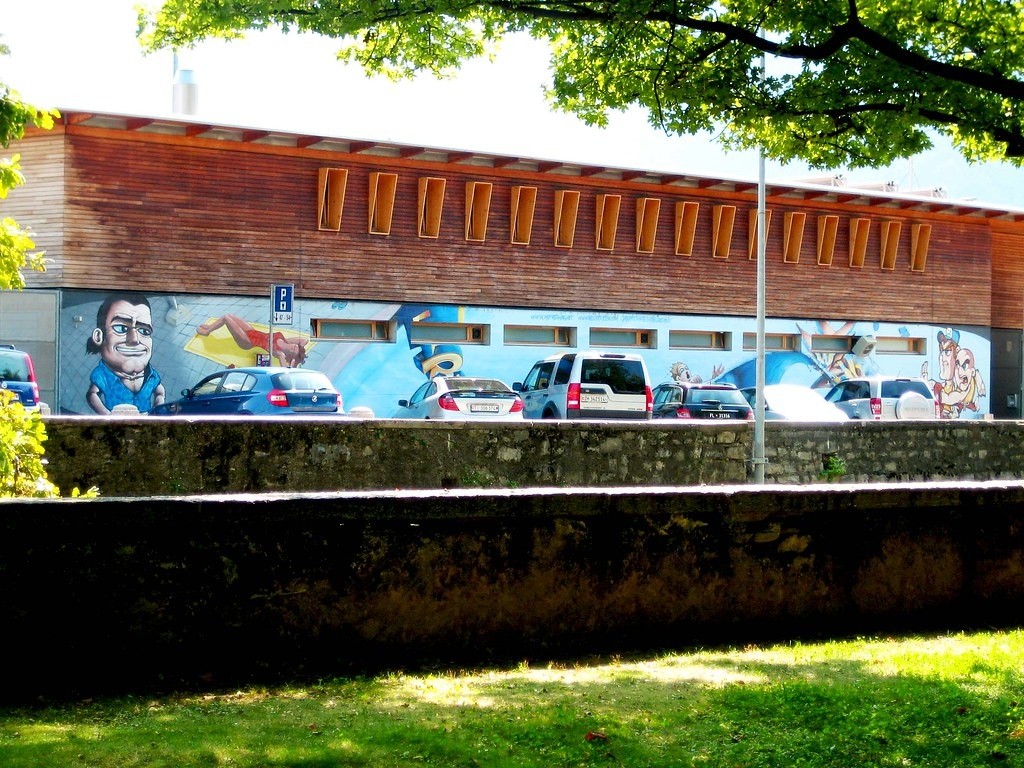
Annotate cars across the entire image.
[150,366,345,416]
[725,384,852,422]
[0,341,42,416]
[392,376,526,420]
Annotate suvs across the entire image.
[652,382,756,420]
[822,376,943,420]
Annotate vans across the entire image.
[512,350,653,420]
